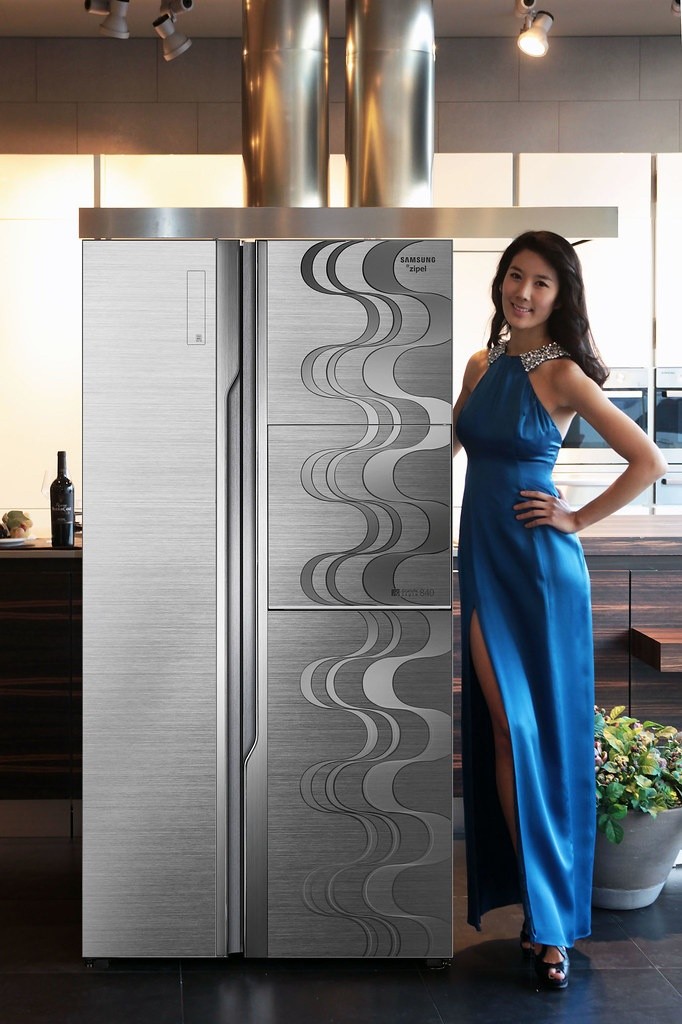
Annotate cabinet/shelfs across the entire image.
[0,549,82,801]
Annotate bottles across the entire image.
[50,451,74,548]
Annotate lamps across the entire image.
[513,0,554,58]
[84,0,130,39]
[152,0,194,61]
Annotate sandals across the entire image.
[536,942,570,989]
[521,915,537,959]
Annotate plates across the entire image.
[0,535,37,547]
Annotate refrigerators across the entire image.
[81,238,454,958]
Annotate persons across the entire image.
[450,227,667,989]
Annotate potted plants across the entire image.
[592,705,682,910]
